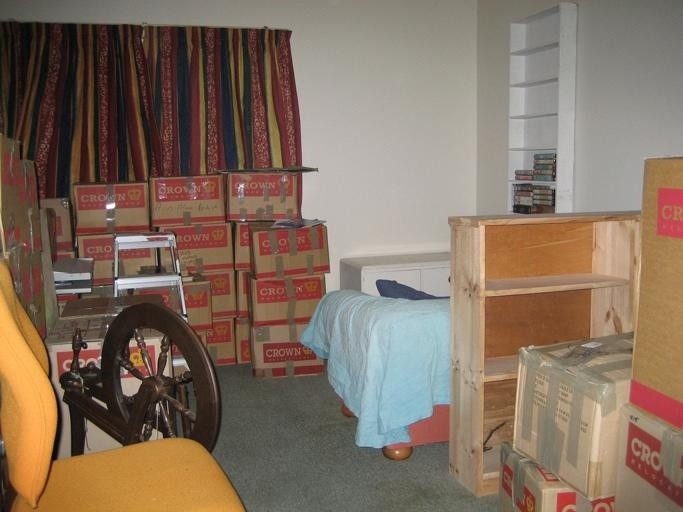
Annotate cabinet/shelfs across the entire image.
[444,213,644,497]
[339,252,453,296]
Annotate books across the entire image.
[513,153,556,214]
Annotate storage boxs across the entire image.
[627,158,683,430]
[149,175,228,226]
[221,172,298,223]
[249,277,327,326]
[72,182,150,235]
[39,199,76,335]
[236,269,251,318]
[233,222,251,270]
[613,403,683,510]
[181,280,215,332]
[160,222,233,272]
[75,233,157,286]
[235,315,247,365]
[0,133,49,340]
[192,317,233,368]
[253,322,325,380]
[54,256,96,291]
[251,221,329,274]
[193,273,238,317]
[57,288,185,366]
[499,443,619,511]
[512,333,636,499]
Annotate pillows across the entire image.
[375,279,450,301]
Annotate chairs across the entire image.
[0,252,247,512]
[299,277,452,461]
